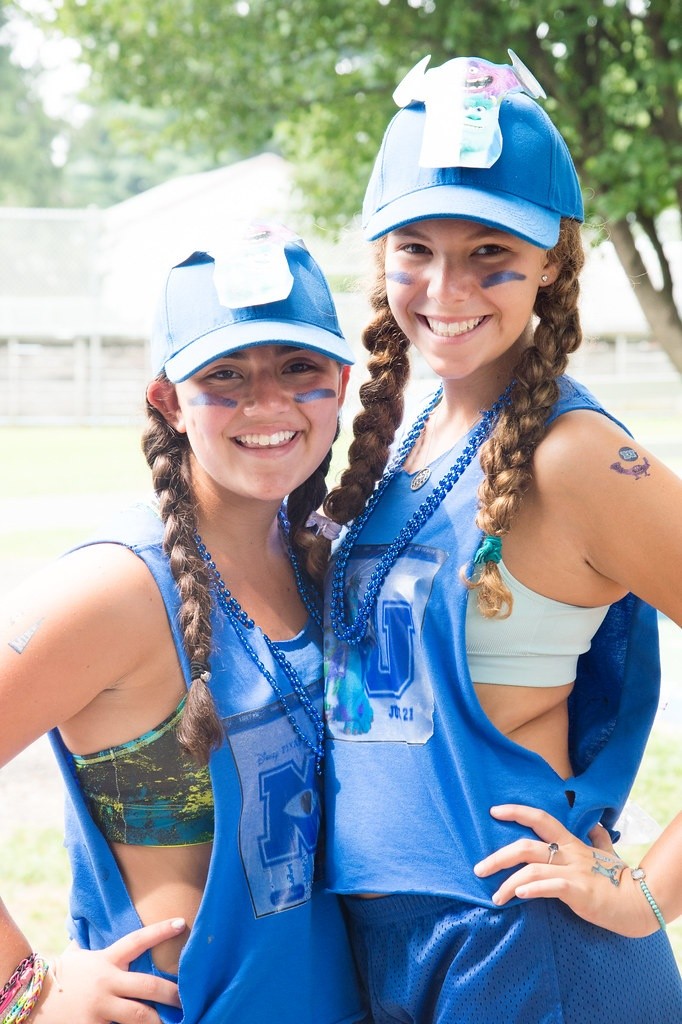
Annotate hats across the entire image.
[362,49,585,251]
[164,228,356,385]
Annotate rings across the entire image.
[547,843,559,863]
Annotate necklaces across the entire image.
[331,378,517,645]
[181,512,327,763]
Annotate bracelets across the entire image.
[0,952,49,1023]
[632,867,666,932]
[0,227,364,1024]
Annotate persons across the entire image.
[318,50,681,1024]
